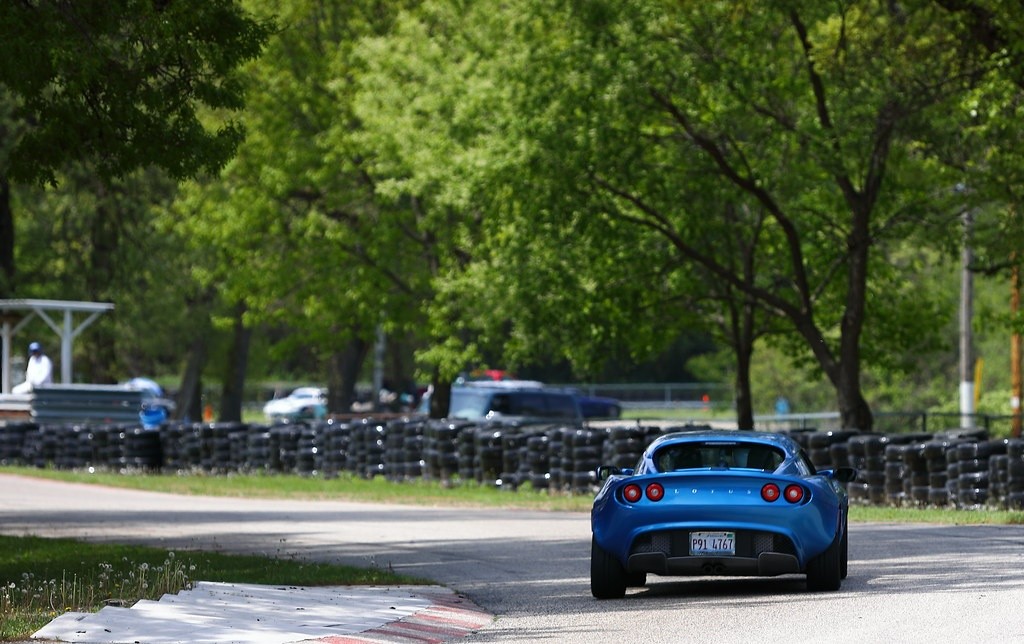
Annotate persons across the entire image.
[11,342,54,394]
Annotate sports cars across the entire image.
[590,429,861,598]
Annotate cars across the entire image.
[263,385,329,424]
[566,386,622,420]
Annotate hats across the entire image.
[29,342,41,352]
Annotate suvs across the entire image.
[412,379,584,425]
